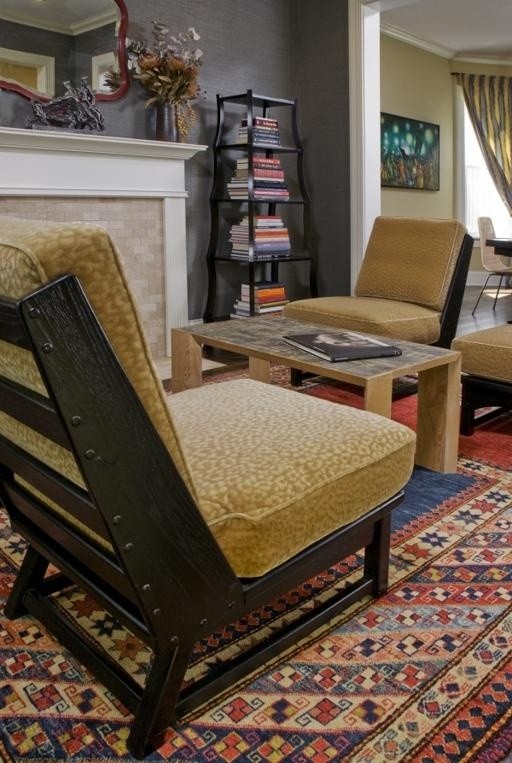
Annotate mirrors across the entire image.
[0,0,131,102]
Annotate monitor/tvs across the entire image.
[380,112,440,191]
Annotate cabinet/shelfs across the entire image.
[205,87,320,356]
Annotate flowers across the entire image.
[122,17,209,142]
[104,66,122,89]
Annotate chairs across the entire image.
[279,215,473,401]
[472,216,511,316]
[1,212,418,761]
[450,321,512,436]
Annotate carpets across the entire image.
[0,357,512,761]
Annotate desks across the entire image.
[170,313,462,487]
[484,238,511,256]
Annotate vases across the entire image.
[151,98,178,140]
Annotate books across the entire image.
[228,116,292,317]
[282,329,404,365]
